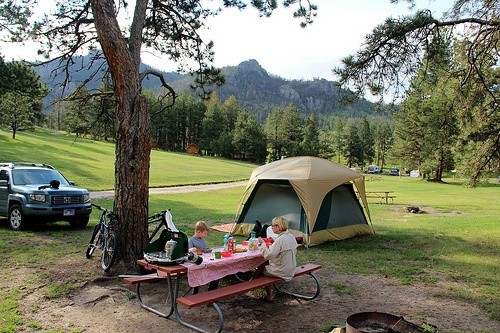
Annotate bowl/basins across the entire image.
[222,251,232,257]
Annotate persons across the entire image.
[187,142,199,151]
[259,216,298,303]
[189,221,220,308]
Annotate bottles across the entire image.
[248,231,259,252]
[185,252,203,265]
[224,235,236,253]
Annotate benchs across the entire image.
[122,273,186,287]
[365,196,397,198]
[175,263,323,309]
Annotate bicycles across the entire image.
[86,203,119,272]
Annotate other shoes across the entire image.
[235,272,253,282]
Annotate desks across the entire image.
[356,191,394,205]
[136,245,320,333]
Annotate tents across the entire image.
[230,155,376,248]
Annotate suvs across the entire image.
[0,161,92,231]
[389,167,399,176]
[367,165,381,174]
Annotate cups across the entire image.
[197,249,202,255]
[215,252,221,259]
